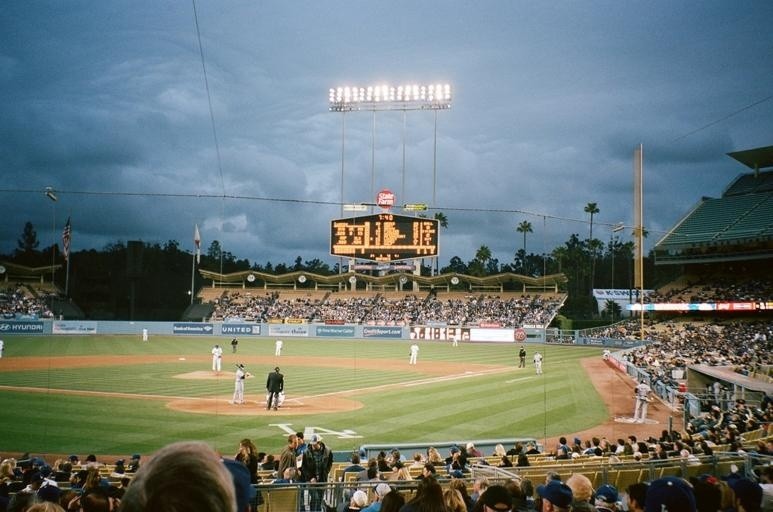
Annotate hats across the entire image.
[0,435,749,510]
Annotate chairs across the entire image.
[522,442,750,511]
[184,287,566,328]
[224,455,522,512]
[1,452,151,511]
[682,405,771,511]
[0,280,87,321]
[608,168,771,394]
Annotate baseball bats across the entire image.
[235,364,246,372]
